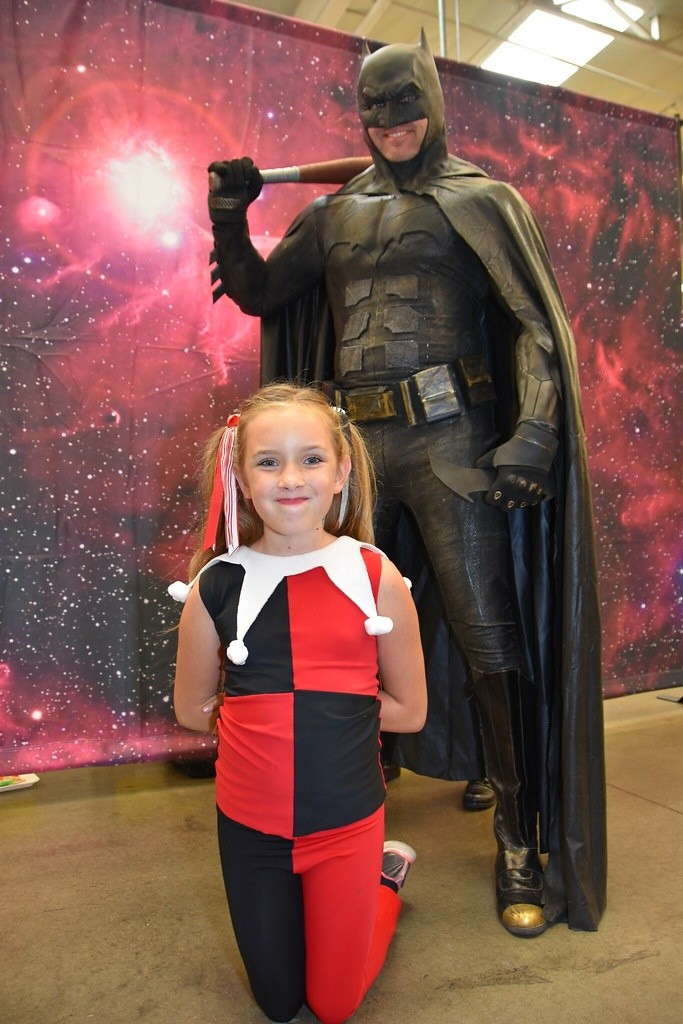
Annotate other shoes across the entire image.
[462,778,497,808]
[496,869,551,935]
[380,840,416,886]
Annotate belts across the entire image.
[327,364,501,426]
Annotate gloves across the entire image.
[207,156,264,223]
[475,421,560,511]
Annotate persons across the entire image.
[173,384,431,1023]
[207,26,606,1024]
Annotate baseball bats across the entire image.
[208,156,375,193]
